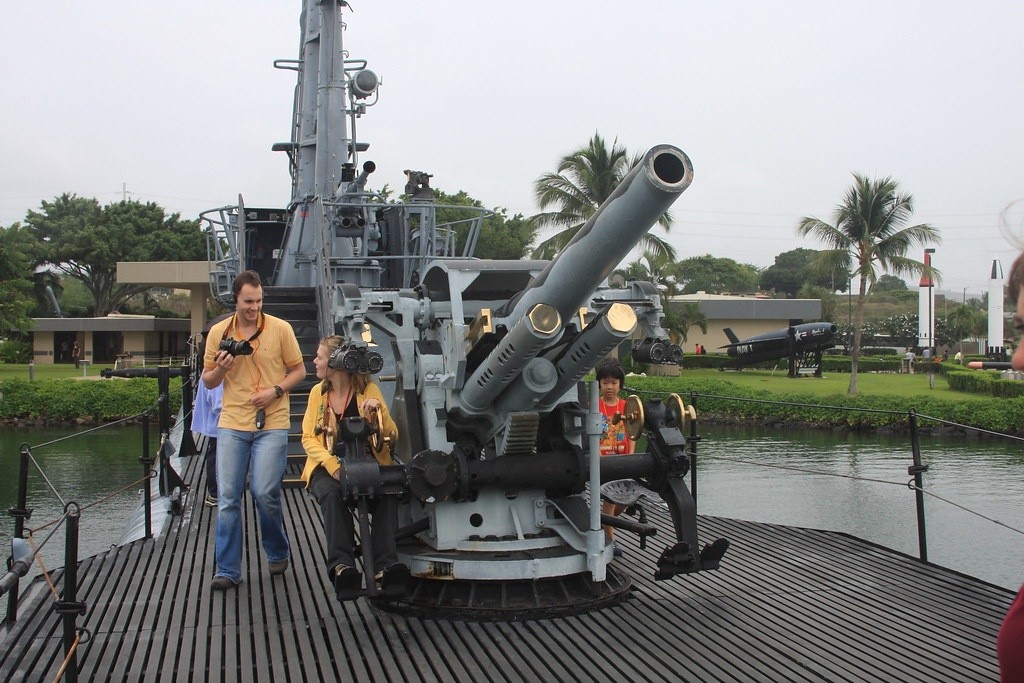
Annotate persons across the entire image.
[71,340,80,369]
[954,350,961,364]
[695,344,700,355]
[60,339,68,361]
[903,346,917,363]
[943,349,948,361]
[591,363,636,559]
[190,373,225,507]
[299,336,400,599]
[701,345,706,355]
[923,346,933,360]
[934,356,941,362]
[997,252,1024,683]
[202,272,306,589]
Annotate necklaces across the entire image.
[327,383,353,418]
[599,396,622,429]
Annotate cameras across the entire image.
[219,337,253,357]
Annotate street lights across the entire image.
[846,274,854,353]
[924,249,935,388]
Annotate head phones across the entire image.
[596,366,625,389]
[229,277,264,304]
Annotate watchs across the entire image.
[274,385,283,399]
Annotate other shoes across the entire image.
[334,563,355,594]
[612,540,623,556]
[375,570,385,582]
[205,493,218,507]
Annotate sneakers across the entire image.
[268,560,289,575]
[211,576,234,589]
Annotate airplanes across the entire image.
[716,315,837,374]
